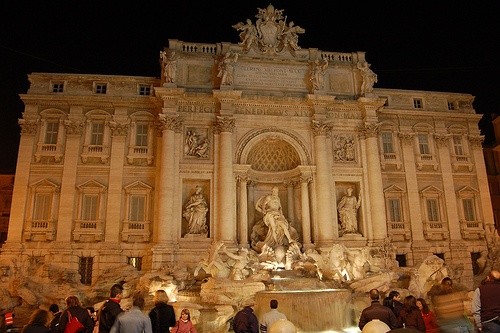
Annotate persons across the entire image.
[0,270,500,333]
[183,130,363,249]
[311,58,329,90]
[357,60,377,98]
[165,51,178,84]
[218,53,239,87]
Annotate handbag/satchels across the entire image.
[64,310,84,333]
